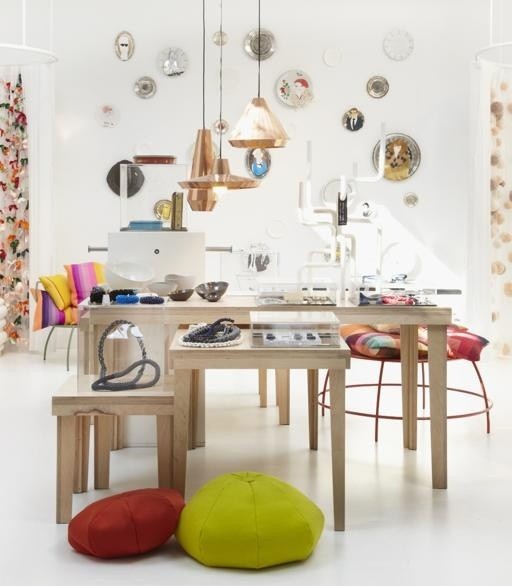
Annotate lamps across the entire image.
[178,0,263,191]
[187,0,220,214]
[226,0,291,148]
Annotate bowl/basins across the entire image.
[168,288,196,301]
[196,280,228,303]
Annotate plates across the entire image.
[382,30,415,60]
[367,76,389,98]
[373,133,419,180]
[276,69,315,106]
[154,199,176,219]
[213,119,230,134]
[323,49,341,67]
[244,27,279,62]
[157,46,188,77]
[135,76,157,99]
[320,177,356,207]
[95,103,123,128]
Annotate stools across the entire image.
[42,321,81,372]
[47,373,175,526]
[315,322,496,444]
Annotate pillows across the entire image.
[358,292,432,306]
[63,261,107,307]
[37,274,73,311]
[338,321,454,360]
[28,287,79,331]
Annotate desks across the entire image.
[86,296,454,495]
[169,324,354,531]
